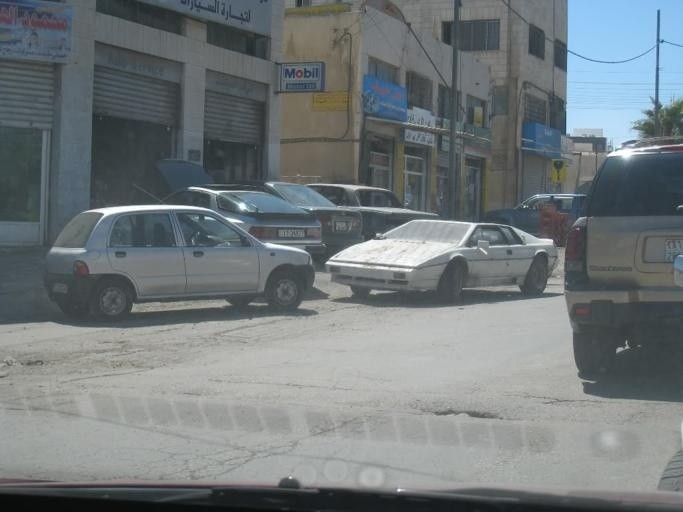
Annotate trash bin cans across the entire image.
[486,208,577,247]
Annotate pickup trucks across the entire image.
[482,192,585,248]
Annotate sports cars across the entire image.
[324,215,560,293]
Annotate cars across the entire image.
[44,204,314,319]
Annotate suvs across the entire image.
[563,136,683,381]
